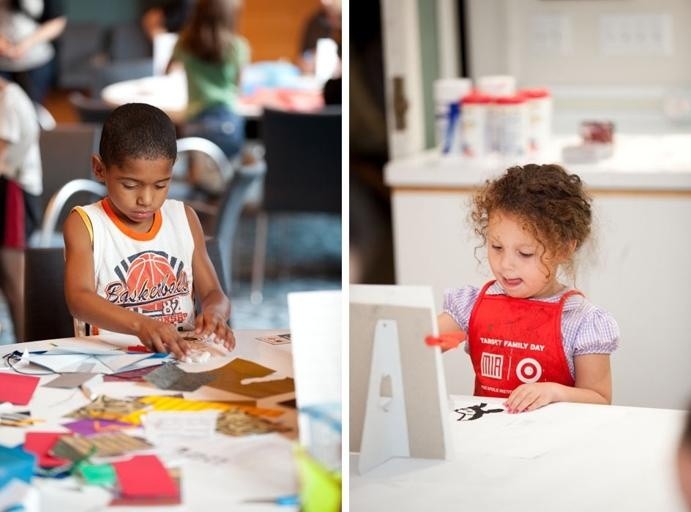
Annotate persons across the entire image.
[435,161,619,414]
[61,100,235,361]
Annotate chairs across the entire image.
[171,106,343,298]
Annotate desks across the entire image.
[353,390,691,510]
[0,329,299,512]
